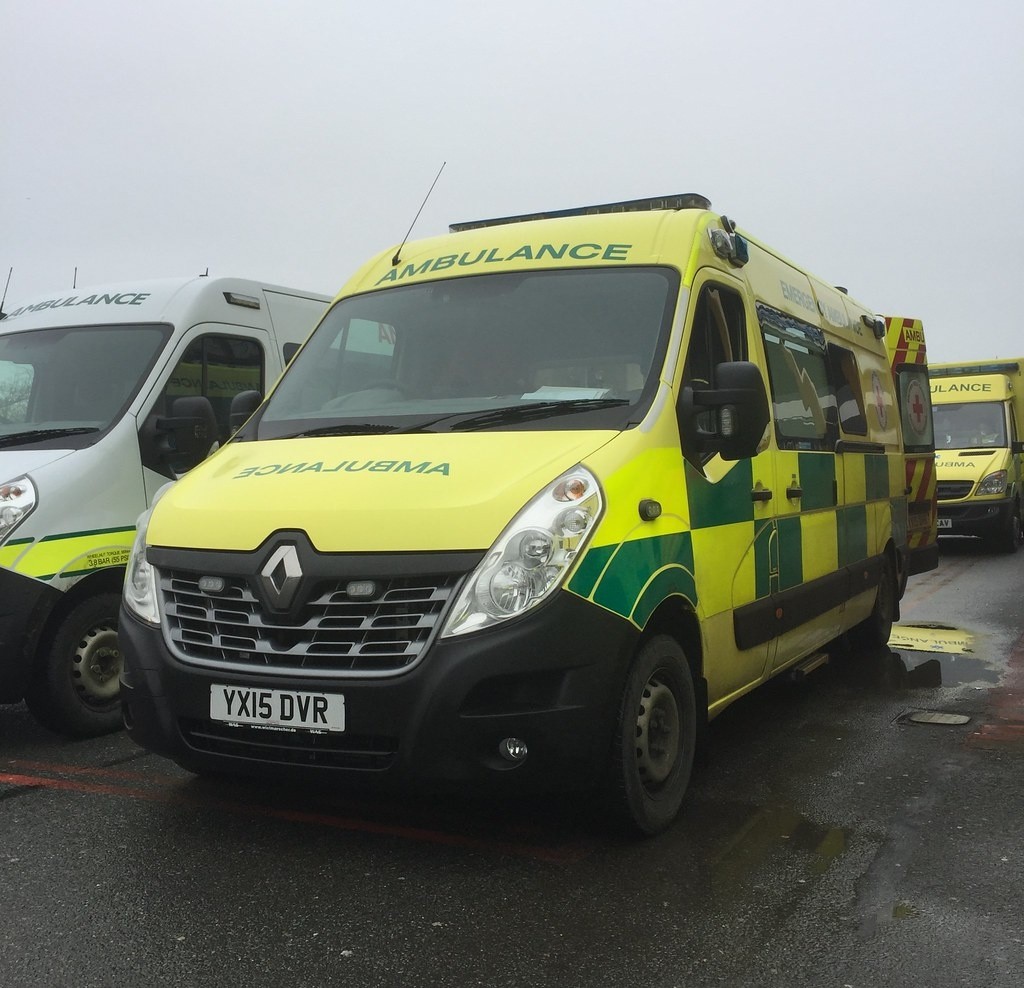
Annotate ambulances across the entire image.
[118,192,942,844]
[924,359,1024,550]
[0,276,397,735]
